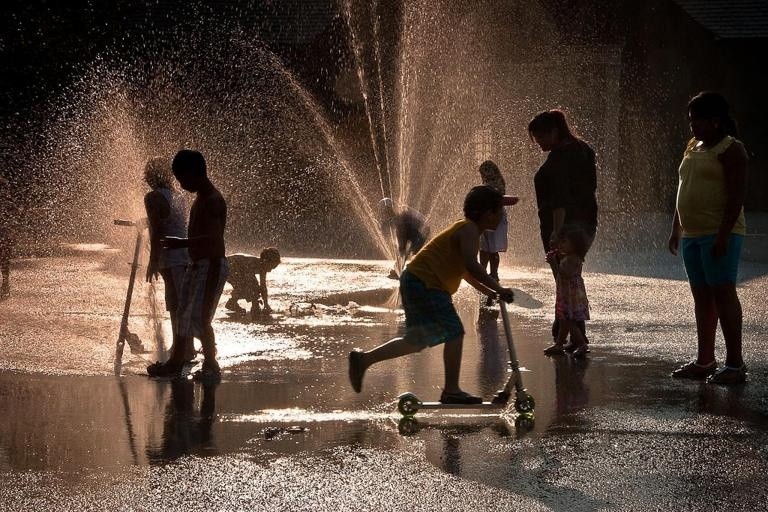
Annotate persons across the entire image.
[146,150,228,383]
[526,109,599,358]
[667,90,753,385]
[477,159,509,284]
[347,186,520,405]
[377,198,432,280]
[224,248,281,314]
[142,156,198,362]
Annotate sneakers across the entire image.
[671,361,717,381]
[348,349,363,394]
[440,391,484,405]
[193,367,221,379]
[147,361,185,376]
[706,364,747,384]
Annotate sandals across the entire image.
[572,343,590,355]
[544,346,564,353]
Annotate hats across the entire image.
[463,186,519,216]
